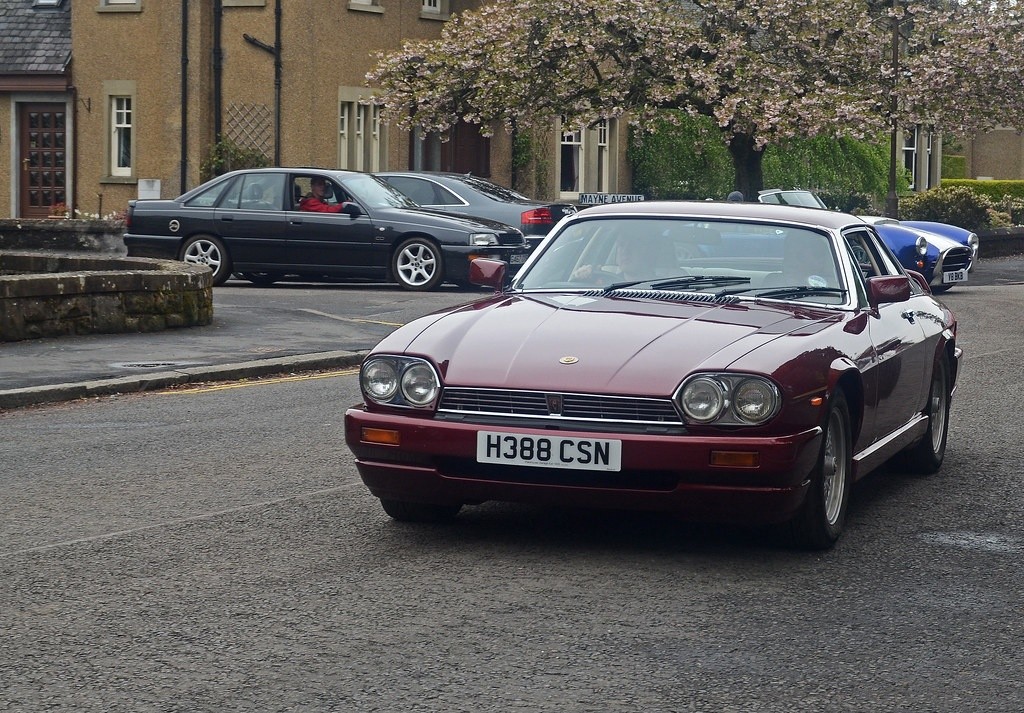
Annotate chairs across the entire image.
[246,183,278,211]
[293,185,303,211]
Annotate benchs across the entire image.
[601,265,780,293]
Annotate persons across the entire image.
[298,176,350,213]
[760,235,829,288]
[570,231,658,288]
[726,190,744,202]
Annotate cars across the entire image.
[663,191,980,296]
[372,171,580,255]
[121,166,532,292]
[345,201,963,558]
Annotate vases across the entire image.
[47,216,68,219]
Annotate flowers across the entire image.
[49,202,72,216]
